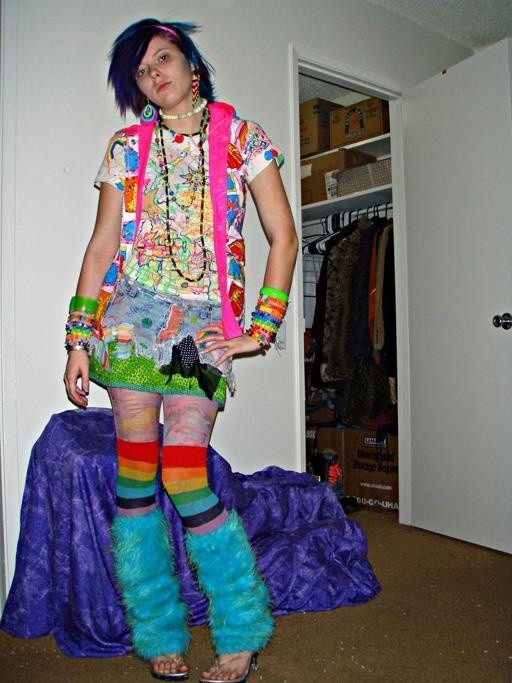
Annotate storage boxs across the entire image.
[299,97,345,159]
[328,97,389,151]
[315,426,397,516]
[300,148,377,206]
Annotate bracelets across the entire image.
[247,288,289,355]
[65,296,96,350]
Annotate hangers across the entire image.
[303,201,390,256]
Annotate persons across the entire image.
[64,19,298,683]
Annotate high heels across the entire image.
[199,653,255,683]
[150,655,189,680]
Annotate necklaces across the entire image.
[159,97,211,282]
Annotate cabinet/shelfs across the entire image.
[301,131,391,210]
